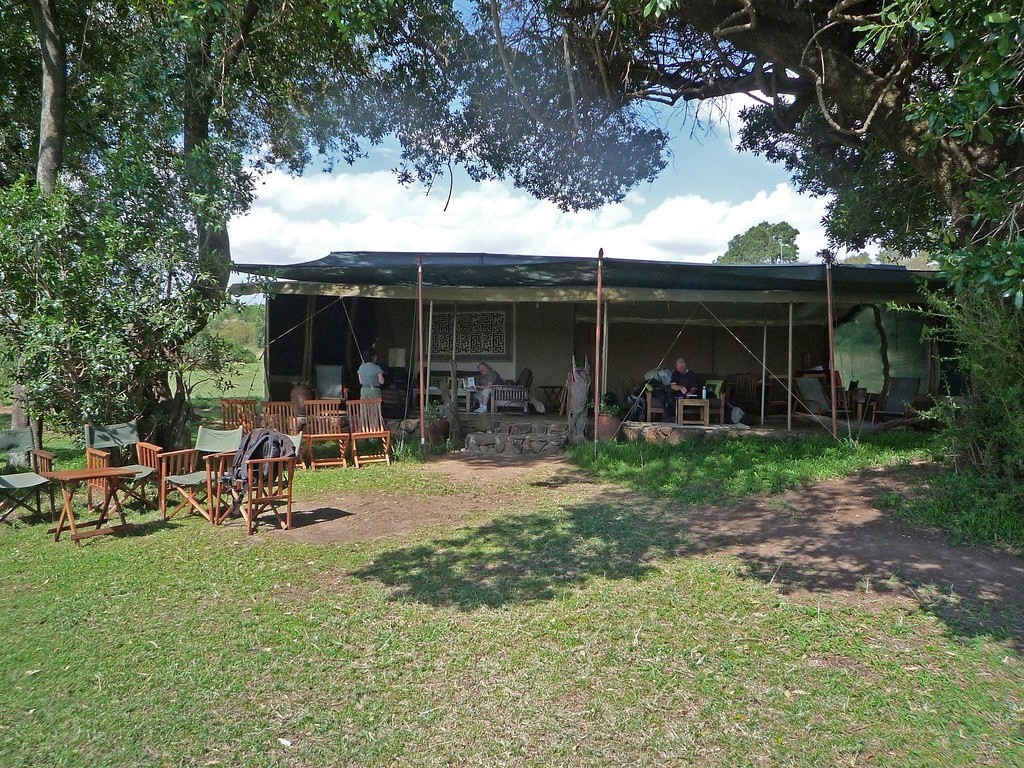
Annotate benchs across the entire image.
[418,371,482,407]
[649,377,726,423]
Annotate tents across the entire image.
[224,252,960,423]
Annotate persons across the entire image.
[667,359,700,421]
[472,363,502,413]
[356,349,384,441]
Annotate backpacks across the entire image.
[230,428,282,483]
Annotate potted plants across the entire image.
[585,396,621,442]
[427,401,449,446]
[290,376,314,417]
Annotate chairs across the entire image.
[347,399,391,469]
[221,399,257,446]
[794,371,860,418]
[731,374,759,424]
[83,419,163,520]
[489,370,535,414]
[0,427,57,524]
[157,426,245,524]
[262,401,309,470]
[769,374,793,416]
[873,375,921,431]
[215,429,303,535]
[302,400,351,470]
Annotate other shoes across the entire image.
[475,408,487,413]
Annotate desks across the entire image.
[412,388,477,413]
[677,398,710,426]
[539,386,564,412]
[39,467,144,546]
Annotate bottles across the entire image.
[702,386,706,399]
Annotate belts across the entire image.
[362,386,380,388]
